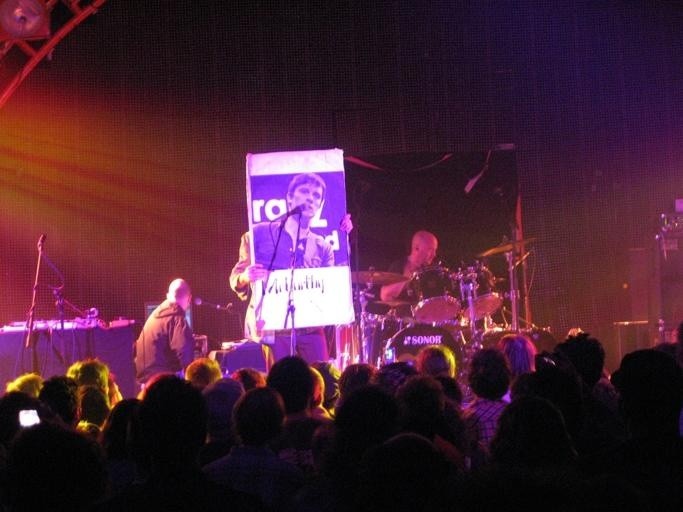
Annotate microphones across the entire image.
[83,307,97,318]
[273,203,306,223]
[33,231,49,248]
[192,296,223,312]
[507,250,533,273]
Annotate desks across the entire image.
[612,319,664,369]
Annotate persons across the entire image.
[250,171,335,273]
[133,277,194,387]
[228,212,354,373]
[0,326,682,511]
[376,228,448,318]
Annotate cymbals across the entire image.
[349,271,408,285]
[478,236,538,258]
[373,300,418,305]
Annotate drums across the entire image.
[455,266,504,321]
[406,265,462,323]
[384,324,464,380]
[470,327,515,354]
